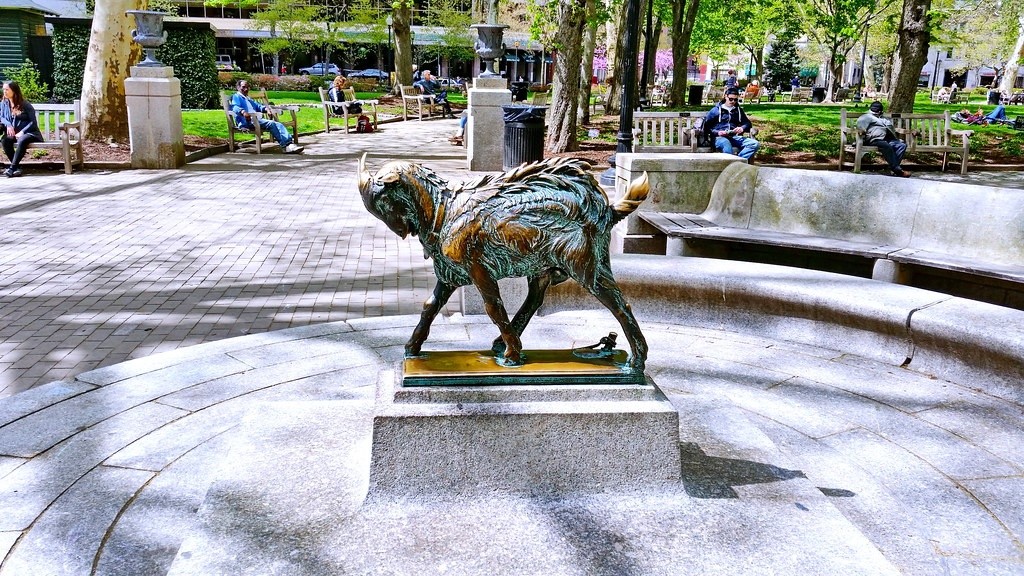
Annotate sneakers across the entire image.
[282,145,305,154]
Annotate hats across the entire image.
[725,86,739,95]
[869,101,884,111]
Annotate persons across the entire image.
[413,70,458,119]
[327,75,362,115]
[663,80,670,98]
[447,83,473,146]
[791,76,800,92]
[519,76,523,82]
[1000,90,1024,106]
[960,105,1006,125]
[412,64,422,81]
[281,66,287,75]
[856,102,912,177]
[747,80,759,96]
[229,80,304,153]
[704,88,760,159]
[0,81,45,176]
[937,81,957,102]
[725,70,736,89]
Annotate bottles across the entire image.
[430,75,436,80]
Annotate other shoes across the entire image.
[897,169,912,178]
[451,142,462,146]
[438,100,446,105]
[448,136,463,142]
[885,167,895,176]
[13,170,24,177]
[3,170,13,177]
[448,114,458,119]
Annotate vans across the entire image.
[215,55,231,66]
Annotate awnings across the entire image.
[500,55,520,61]
[521,55,537,62]
[538,56,554,63]
[800,67,818,77]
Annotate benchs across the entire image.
[637,161,1024,284]
[220,88,300,154]
[399,83,445,121]
[932,90,971,104]
[319,86,380,135]
[651,87,672,108]
[999,93,1024,106]
[839,108,974,175]
[0,100,84,174]
[631,107,759,164]
[515,90,549,105]
[702,83,888,104]
[590,94,608,114]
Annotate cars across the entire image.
[438,78,463,93]
[348,68,388,81]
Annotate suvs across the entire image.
[298,62,341,75]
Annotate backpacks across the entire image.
[357,115,373,133]
[693,117,711,147]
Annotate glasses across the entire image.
[727,96,738,101]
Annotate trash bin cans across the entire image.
[511,82,529,102]
[501,104,549,173]
[812,87,826,103]
[988,92,1001,105]
[688,85,705,105]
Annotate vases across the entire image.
[125,10,171,67]
[470,24,510,79]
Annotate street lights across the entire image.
[514,39,521,81]
[410,31,415,50]
[386,15,392,93]
[930,47,941,98]
[853,14,873,102]
